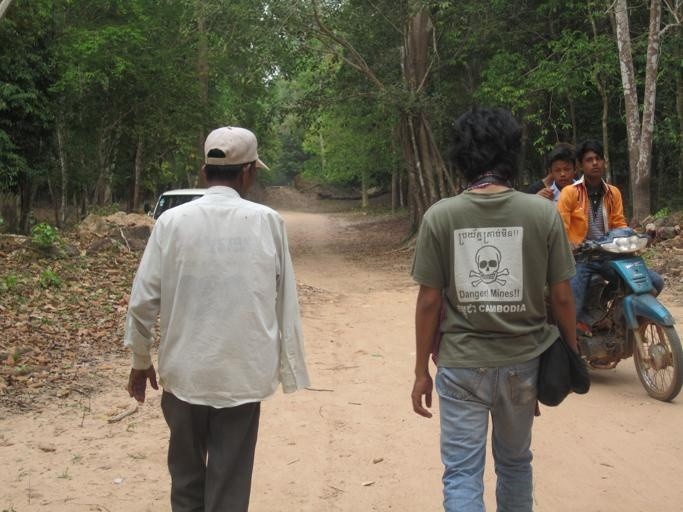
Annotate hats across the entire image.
[203,125,271,172]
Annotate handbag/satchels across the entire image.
[536,335,591,408]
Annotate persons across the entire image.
[410,104,578,512]
[556,139,630,337]
[523,142,577,194]
[536,145,578,207]
[120,124,310,511]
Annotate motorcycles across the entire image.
[568,222,683,403]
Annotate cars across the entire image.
[143,187,208,219]
